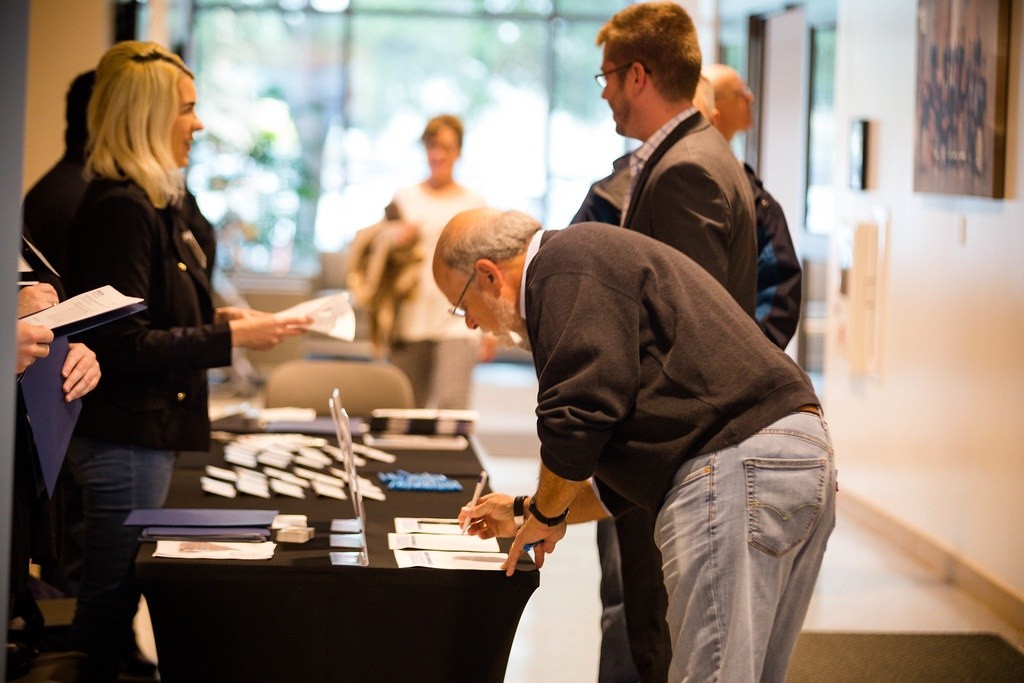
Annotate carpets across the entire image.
[783,630,1024,683]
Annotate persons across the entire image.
[431,205,841,683]
[22,67,101,300]
[595,0,757,326]
[915,34,990,193]
[5,220,102,676]
[65,39,315,683]
[567,73,803,351]
[374,112,501,412]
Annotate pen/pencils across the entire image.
[460,470,488,535]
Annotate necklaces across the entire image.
[428,177,454,189]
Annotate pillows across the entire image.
[321,253,346,289]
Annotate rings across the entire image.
[52,302,57,307]
[482,521,487,529]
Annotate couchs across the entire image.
[304,274,375,360]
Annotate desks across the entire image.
[132,415,540,683]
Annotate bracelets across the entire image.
[512,494,529,533]
[527,493,572,528]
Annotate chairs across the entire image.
[265,358,414,414]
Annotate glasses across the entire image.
[448,257,497,316]
[594,64,651,87]
